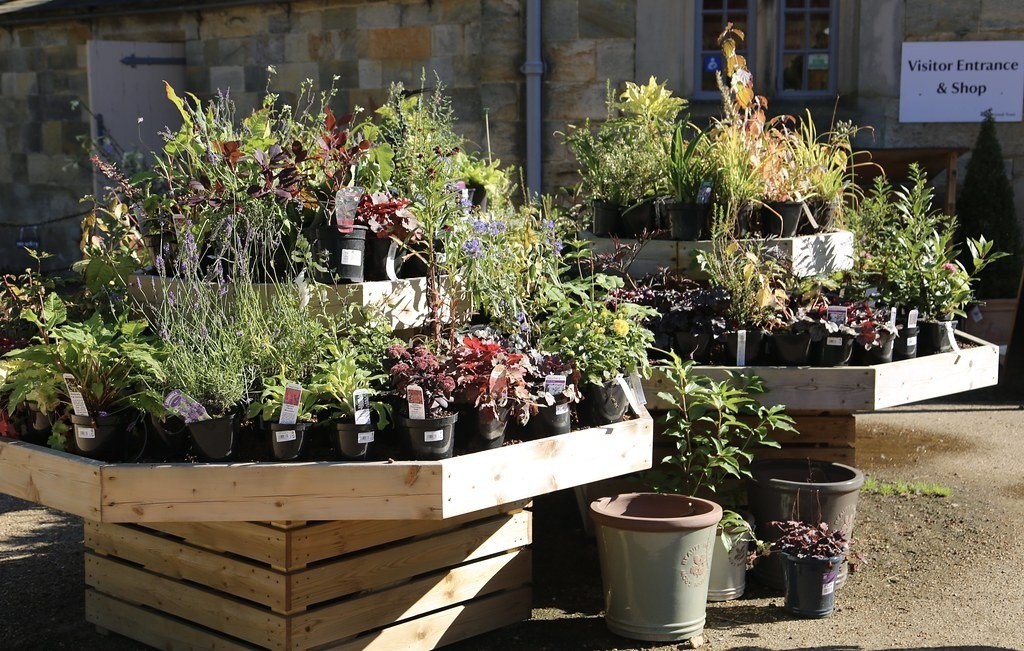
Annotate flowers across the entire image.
[135,211,258,412]
[559,286,653,385]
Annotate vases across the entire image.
[750,459,863,590]
[589,492,723,642]
[185,415,240,464]
[581,375,631,426]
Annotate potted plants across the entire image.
[304,117,390,284]
[16,262,129,461]
[254,375,310,462]
[613,257,763,367]
[411,171,455,277]
[564,77,856,239]
[879,249,921,361]
[396,361,459,460]
[521,350,580,439]
[314,349,390,459]
[459,345,514,451]
[950,106,1022,363]
[918,235,1008,355]
[123,338,188,465]
[851,298,901,367]
[251,145,308,284]
[652,349,799,602]
[4,343,74,449]
[363,150,411,278]
[768,520,849,620]
[762,297,852,366]
